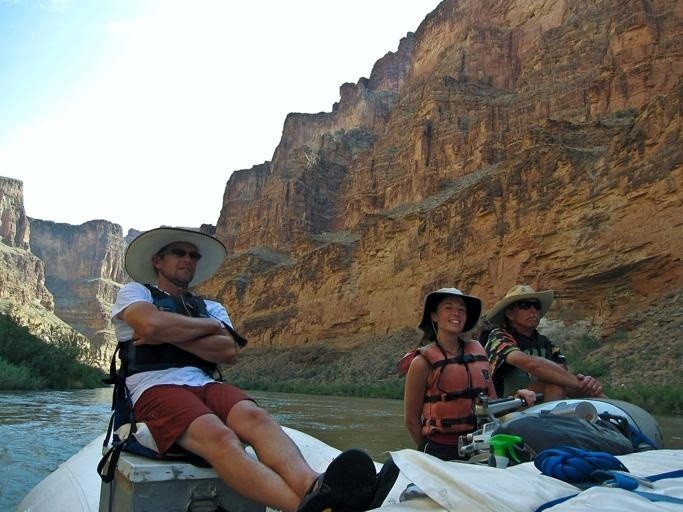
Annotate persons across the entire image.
[482,282,608,412]
[108,225,402,511]
[396,287,538,460]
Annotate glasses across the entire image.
[508,300,543,311]
[156,248,202,260]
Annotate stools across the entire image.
[95,444,265,512]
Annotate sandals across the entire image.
[297,449,400,512]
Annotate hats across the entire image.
[484,283,556,330]
[416,285,483,343]
[123,223,228,289]
